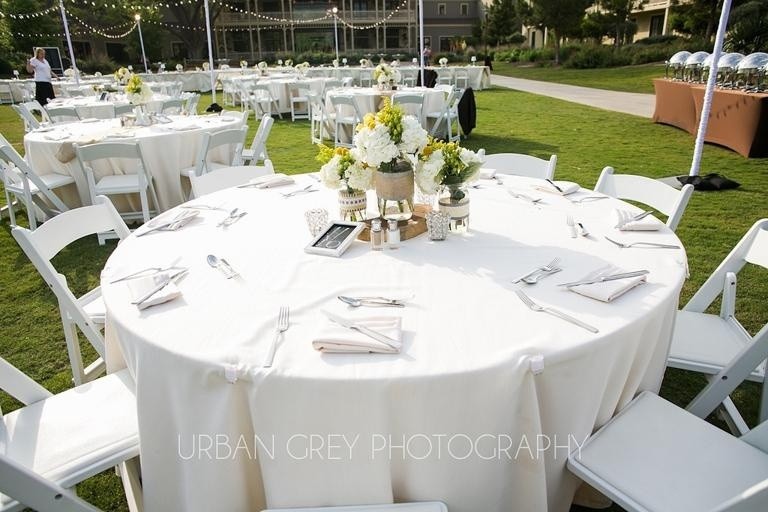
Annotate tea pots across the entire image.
[220,109,235,121]
[379,82,388,92]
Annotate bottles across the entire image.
[438,185,450,200]
[458,184,470,199]
[371,221,383,251]
[386,220,400,250]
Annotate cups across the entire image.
[416,188,437,207]
[425,212,449,241]
[305,210,329,237]
[40,122,50,130]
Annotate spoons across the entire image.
[180,200,225,211]
[338,296,407,308]
[497,180,503,185]
[285,184,314,196]
[206,254,232,279]
[522,268,562,285]
[567,196,609,203]
[517,193,542,203]
[576,222,590,236]
[109,256,180,284]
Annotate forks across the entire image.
[508,189,541,210]
[567,216,578,238]
[264,307,290,367]
[515,290,598,333]
[512,255,561,284]
[281,189,320,197]
[605,236,680,250]
[470,183,481,189]
[221,258,241,282]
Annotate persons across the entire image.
[26,47,61,116]
[140,55,152,73]
[423,44,431,67]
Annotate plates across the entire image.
[32,127,56,133]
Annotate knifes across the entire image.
[238,181,265,189]
[136,212,199,237]
[545,178,563,193]
[557,270,648,287]
[131,270,189,306]
[318,306,402,350]
[216,207,238,228]
[615,210,654,228]
[225,213,247,227]
